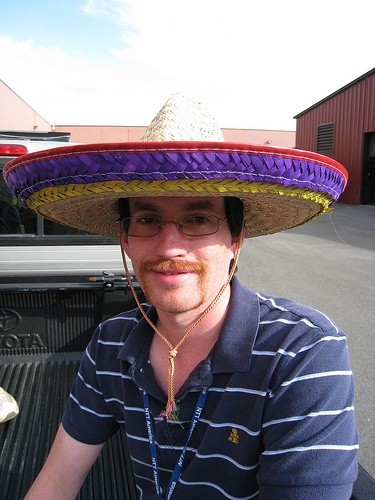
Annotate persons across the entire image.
[0,90,363,500]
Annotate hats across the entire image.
[1,88,349,241]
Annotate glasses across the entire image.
[119,213,228,237]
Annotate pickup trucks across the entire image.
[0,140,375,500]
